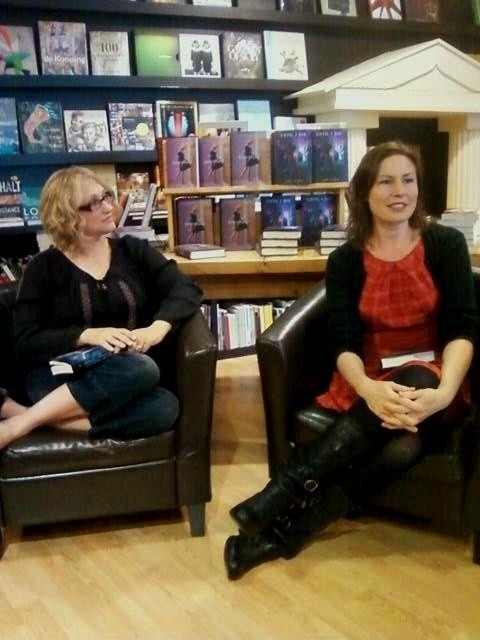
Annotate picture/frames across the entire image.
[155,98,200,148]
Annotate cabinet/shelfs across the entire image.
[1,2,480,292]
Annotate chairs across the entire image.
[0,277,218,564]
[254,270,479,563]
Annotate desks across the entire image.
[157,244,480,343]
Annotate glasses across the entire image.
[78,190,114,212]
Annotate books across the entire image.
[440,209,477,245]
[379,351,444,370]
[1,168,171,282]
[0,98,20,154]
[200,302,286,351]
[108,104,156,151]
[0,21,182,76]
[222,32,264,79]
[16,101,66,153]
[155,0,440,23]
[155,101,199,145]
[262,31,308,81]
[178,34,222,78]
[159,100,349,260]
[63,110,110,152]
[48,344,116,376]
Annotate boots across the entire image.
[224,480,361,580]
[229,414,367,535]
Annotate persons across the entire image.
[201,41,213,76]
[176,148,190,184]
[222,139,478,582]
[324,144,335,167]
[228,39,260,63]
[186,206,205,245]
[209,145,222,184]
[191,41,202,75]
[67,112,107,152]
[239,142,259,181]
[0,167,208,452]
[287,145,298,175]
[230,205,248,242]
[279,48,303,74]
